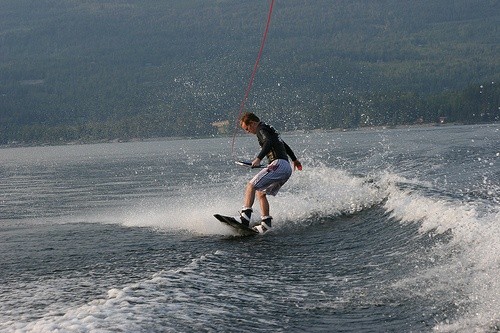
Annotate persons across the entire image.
[230,112,302,233]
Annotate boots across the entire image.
[252,216,273,233]
[230,208,253,226]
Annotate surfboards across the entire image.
[214,213,259,235]
